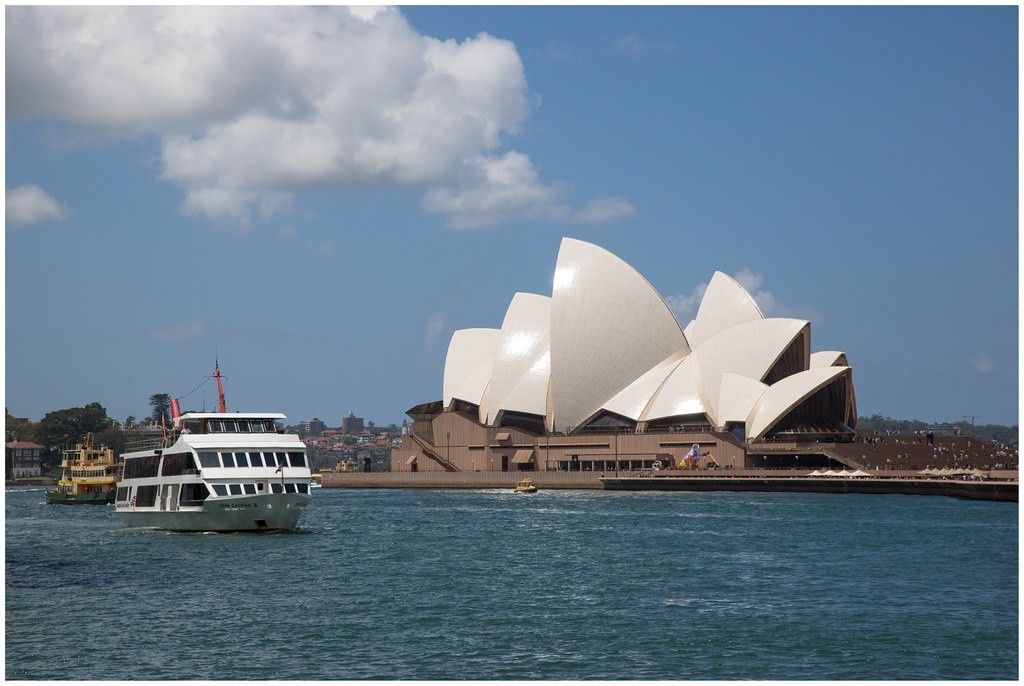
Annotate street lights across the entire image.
[447,432,450,471]
[615,427,618,478]
[546,428,549,471]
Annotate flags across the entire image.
[162,420,167,439]
[275,465,281,474]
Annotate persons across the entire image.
[130,496,136,506]
[852,431,1018,470]
[662,465,681,470]
[824,475,985,481]
[670,424,684,433]
[181,426,191,434]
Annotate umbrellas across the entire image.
[918,469,985,478]
[807,470,866,478]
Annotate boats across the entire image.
[113,347,312,533]
[513,478,538,494]
[311,458,361,484]
[44,432,123,505]
